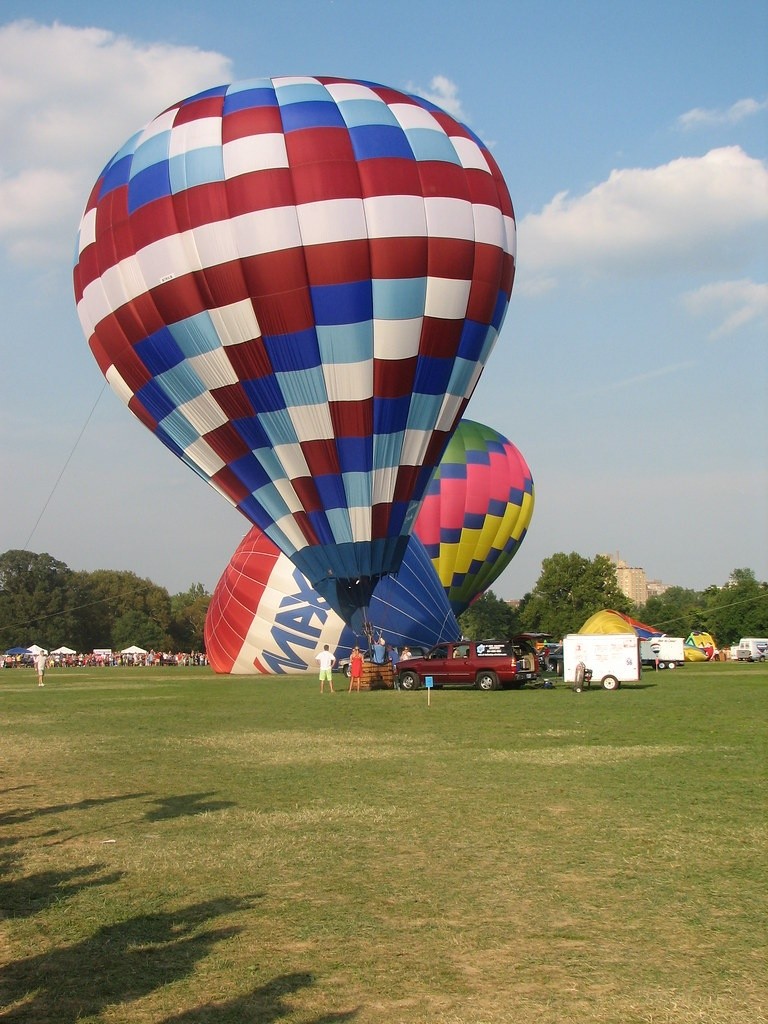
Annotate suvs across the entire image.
[337,644,432,678]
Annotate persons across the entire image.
[33,650,50,687]
[371,638,386,664]
[314,645,336,695]
[542,645,552,673]
[386,645,402,692]
[401,646,412,660]
[347,645,365,694]
[0,651,209,670]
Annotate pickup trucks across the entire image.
[396,633,553,691]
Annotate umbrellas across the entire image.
[4,647,32,654]
[50,646,77,655]
[121,646,147,653]
[21,645,48,656]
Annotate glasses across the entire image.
[404,649,408,650]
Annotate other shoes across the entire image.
[331,690,336,692]
[320,691,324,693]
[38,683,46,687]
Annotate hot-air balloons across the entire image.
[205,421,536,676]
[577,609,731,662]
[74,73,518,689]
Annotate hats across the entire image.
[380,638,385,645]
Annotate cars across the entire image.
[538,643,563,670]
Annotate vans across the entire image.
[739,639,768,662]
